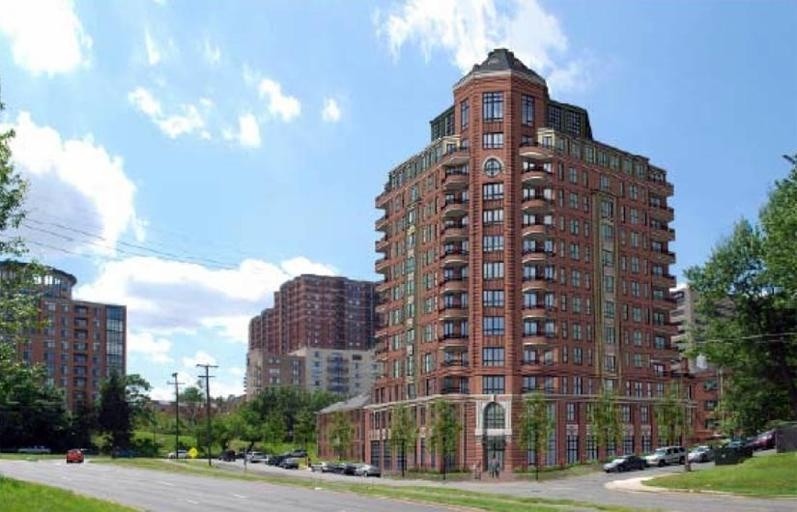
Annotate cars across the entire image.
[17,445,142,465]
[217,448,383,477]
[601,426,774,476]
[168,449,189,460]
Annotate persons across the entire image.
[488,454,501,479]
[471,460,481,481]
